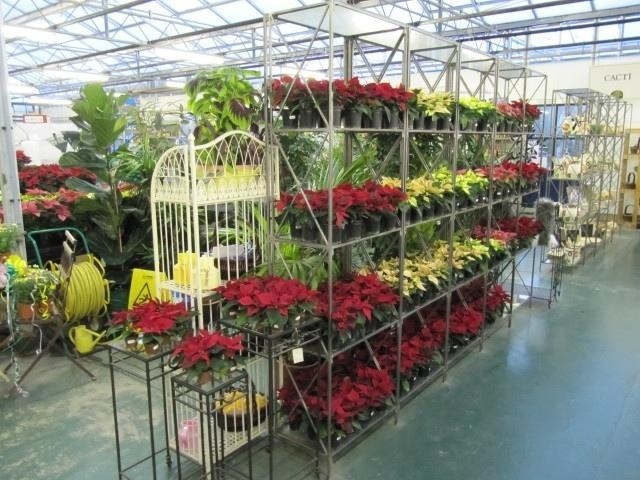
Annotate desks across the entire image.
[99,309,323,480]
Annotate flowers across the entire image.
[0,149,103,248]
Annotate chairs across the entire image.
[1,228,100,398]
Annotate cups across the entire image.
[181,419,197,450]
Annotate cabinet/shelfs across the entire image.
[272,122,545,480]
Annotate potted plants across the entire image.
[111,275,325,432]
[186,65,274,275]
[273,76,543,447]
[8,275,44,323]
